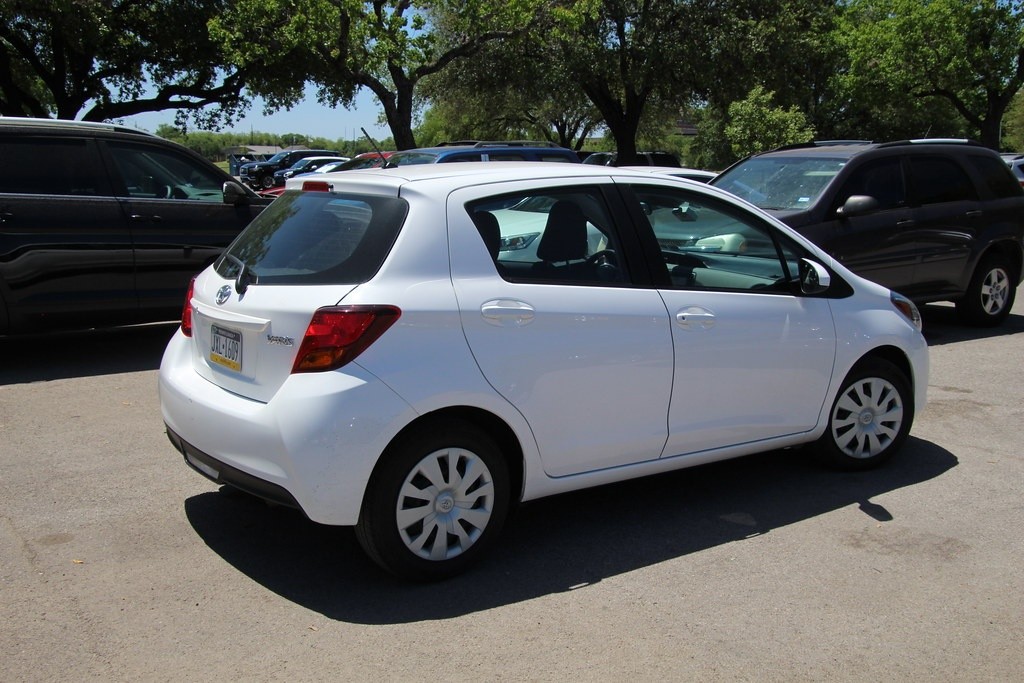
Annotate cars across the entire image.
[239,150,341,192]
[998,152,1024,188]
[272,156,350,187]
[582,152,681,168]
[259,151,396,197]
[486,168,811,265]
[293,162,345,177]
[158,160,928,581]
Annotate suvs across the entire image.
[327,139,581,212]
[647,139,1024,327]
[0,116,374,346]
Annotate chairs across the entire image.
[471,210,510,279]
[532,201,600,281]
